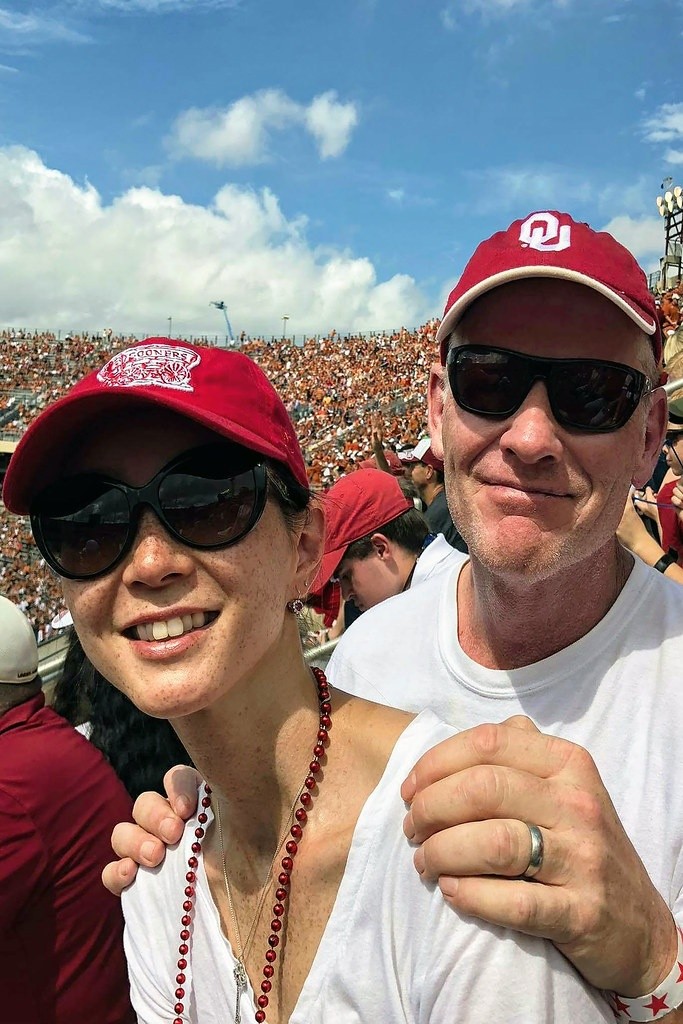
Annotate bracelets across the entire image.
[325,632,330,642]
[653,545,679,572]
[603,923,683,1022]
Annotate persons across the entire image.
[98,211,683,1024]
[0,339,620,1024]
[53,621,196,803]
[0,596,144,1024]
[615,278,682,587]
[308,468,470,615]
[0,328,473,642]
[399,436,468,555]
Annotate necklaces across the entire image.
[214,717,317,1023]
[173,665,334,1024]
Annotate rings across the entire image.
[525,823,545,876]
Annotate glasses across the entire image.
[447,344,652,433]
[665,431,683,446]
[29,439,267,582]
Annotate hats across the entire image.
[52,609,73,629]
[668,397,683,430]
[402,438,443,472]
[359,450,400,474]
[3,336,309,515]
[309,468,409,593]
[0,595,38,683]
[436,211,662,384]
